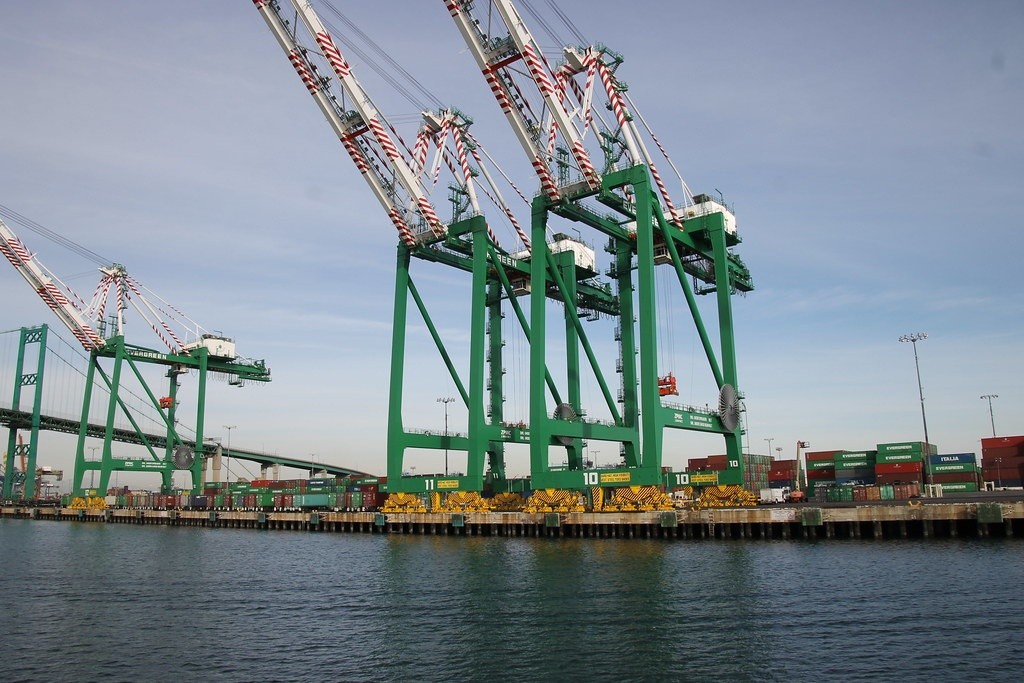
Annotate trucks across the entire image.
[759,488,788,504]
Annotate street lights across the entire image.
[764,438,774,457]
[88,447,100,489]
[436,397,455,477]
[223,425,236,491]
[979,394,999,437]
[899,332,934,486]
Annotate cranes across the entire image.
[447,0,757,501]
[251,0,619,508]
[0,205,273,510]
